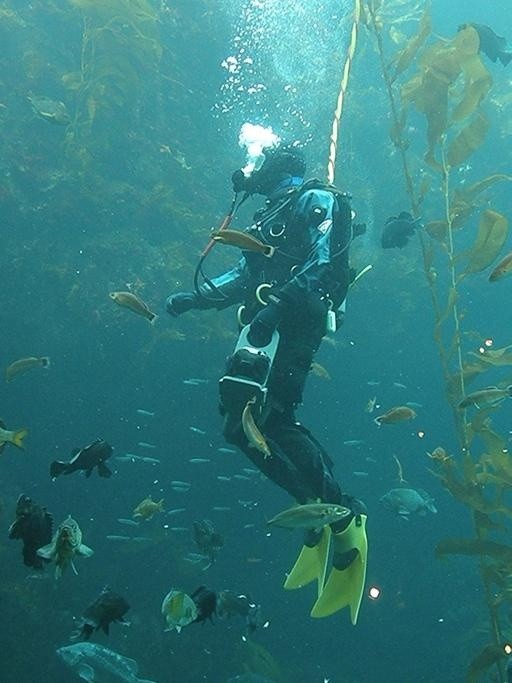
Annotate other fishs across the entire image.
[1,377,285,677]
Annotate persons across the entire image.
[165,141,368,625]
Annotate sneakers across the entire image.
[301,526,325,549]
[327,493,369,570]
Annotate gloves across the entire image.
[249,305,284,342]
[165,290,198,317]
